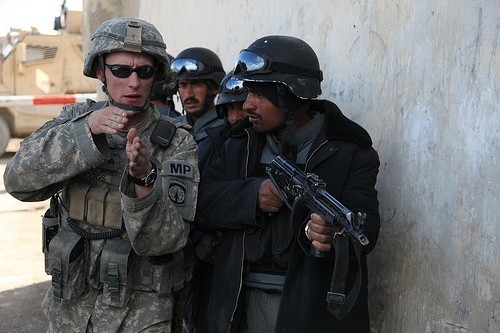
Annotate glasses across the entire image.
[220,70,246,93]
[104,64,155,79]
[233,49,321,80]
[169,58,225,77]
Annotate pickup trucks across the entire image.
[0,93,98,157]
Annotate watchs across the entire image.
[131,165,156,187]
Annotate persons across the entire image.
[4,17,200,333]
[148,46,249,333]
[194,35,381,333]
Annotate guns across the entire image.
[260,153,370,256]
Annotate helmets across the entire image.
[149,53,178,100]
[231,35,322,100]
[83,17,169,82]
[176,47,226,86]
[214,69,249,118]
